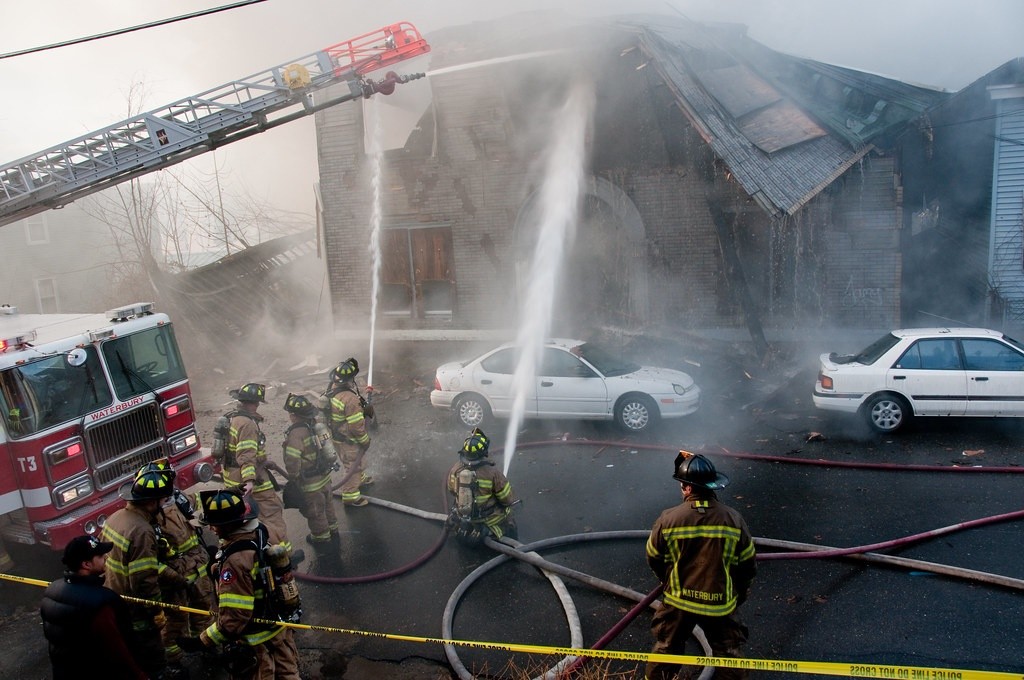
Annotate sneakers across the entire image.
[165,663,189,680]
[330,529,339,538]
[342,496,369,507]
[306,533,332,544]
[289,548,304,561]
[360,477,374,485]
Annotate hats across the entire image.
[62,536,114,568]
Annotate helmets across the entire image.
[230,383,268,404]
[334,358,359,379]
[118,463,176,501]
[200,491,260,524]
[283,394,319,419]
[462,430,490,460]
[673,453,730,489]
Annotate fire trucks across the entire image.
[1,20,432,567]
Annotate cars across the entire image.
[812,326,1024,440]
[430,338,702,433]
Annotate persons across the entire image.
[220,384,291,569]
[283,393,341,549]
[324,357,373,507]
[645,452,757,680]
[447,428,518,556]
[39,462,303,680]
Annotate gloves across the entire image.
[175,634,207,653]
